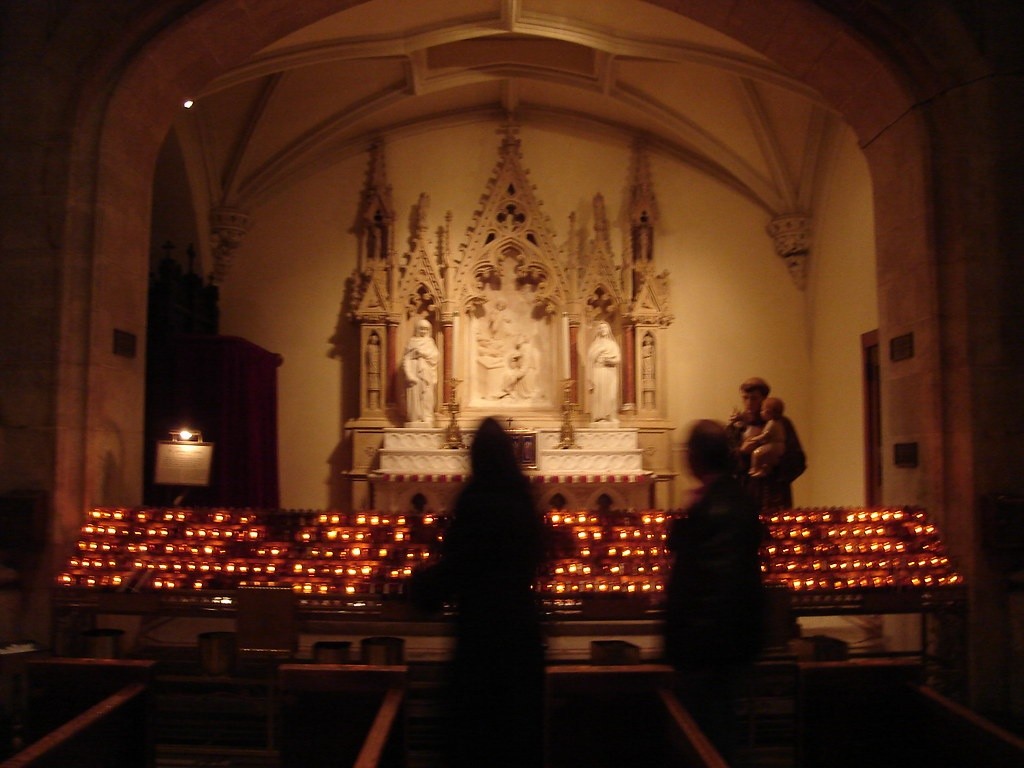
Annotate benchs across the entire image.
[794,658,1024,768]
[546,665,732,767]
[0,655,165,768]
[274,663,412,768]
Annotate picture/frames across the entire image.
[154,441,216,487]
[113,329,138,359]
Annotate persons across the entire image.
[401,319,440,422]
[723,378,807,517]
[443,419,546,768]
[585,321,621,422]
[657,421,768,768]
[748,397,785,478]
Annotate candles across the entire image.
[58,505,960,599]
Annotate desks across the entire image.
[340,471,679,511]
